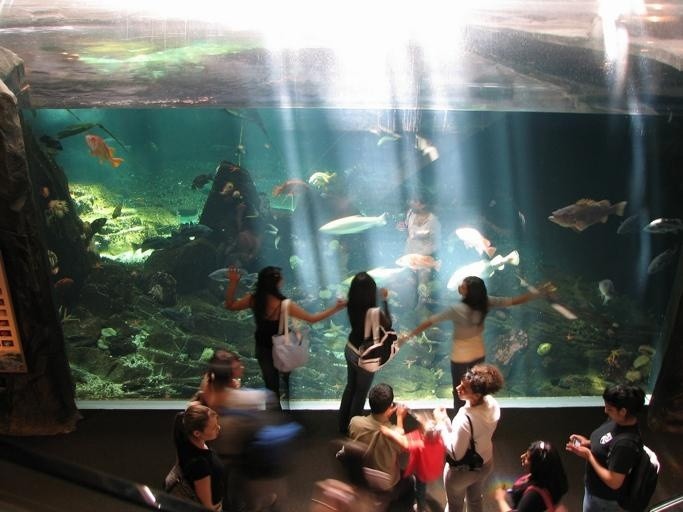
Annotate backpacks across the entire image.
[617,442,660,512]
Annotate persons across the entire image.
[160,349,658,512]
[336,270,394,436]
[396,274,557,416]
[396,185,441,274]
[224,262,348,402]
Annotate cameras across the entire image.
[573,437,581,447]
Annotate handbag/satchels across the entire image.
[271,329,310,373]
[357,328,403,373]
[442,450,487,470]
